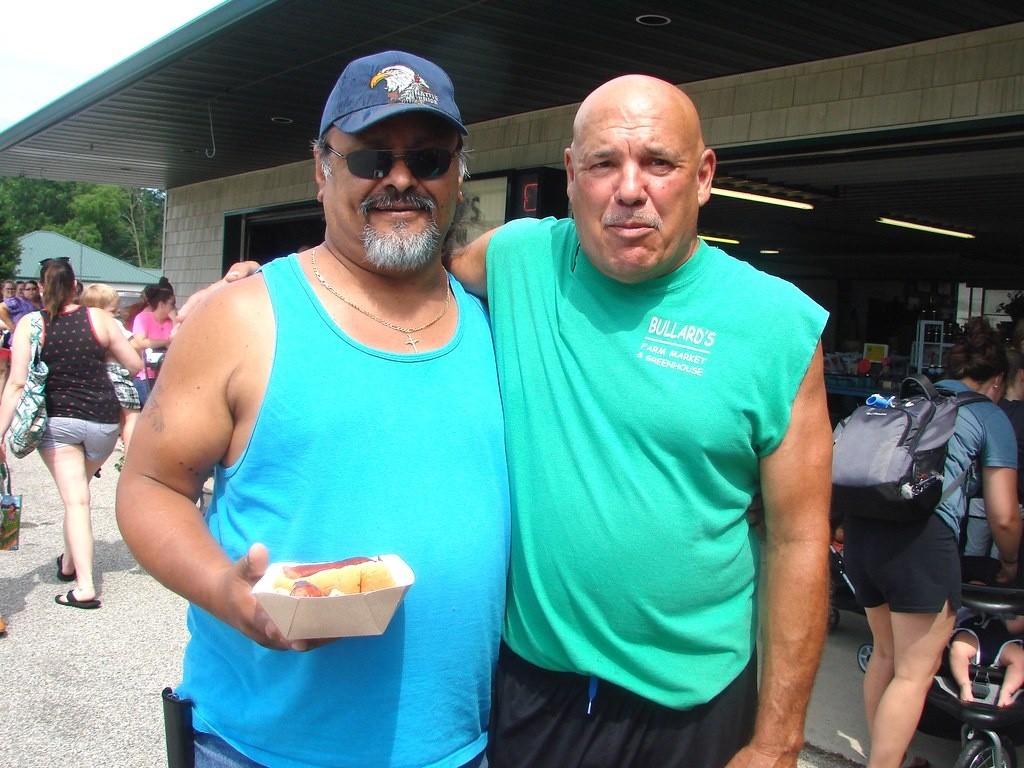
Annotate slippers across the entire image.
[50,589,101,609]
[51,549,75,582]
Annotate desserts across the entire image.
[273,555,398,598]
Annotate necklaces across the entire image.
[305,233,450,356]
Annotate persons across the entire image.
[166,70,835,768]
[11,282,39,373]
[133,273,189,414]
[960,335,1023,560]
[952,576,1024,708]
[1,250,146,612]
[70,278,86,306]
[0,280,47,363]
[77,284,151,477]
[119,289,156,338]
[833,519,848,561]
[102,44,519,765]
[1005,317,1024,354]
[820,313,1024,768]
[1,393,8,638]
[0,281,18,379]
[37,282,44,294]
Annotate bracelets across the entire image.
[997,550,1020,566]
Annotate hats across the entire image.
[316,48,469,147]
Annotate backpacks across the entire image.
[816,358,994,524]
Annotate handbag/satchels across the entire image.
[0,460,24,556]
[141,347,168,368]
[6,321,57,465]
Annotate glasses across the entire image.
[22,286,38,291]
[39,256,74,265]
[17,287,22,291]
[316,142,462,184]
[4,287,14,291]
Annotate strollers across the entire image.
[827,533,1023,768]
